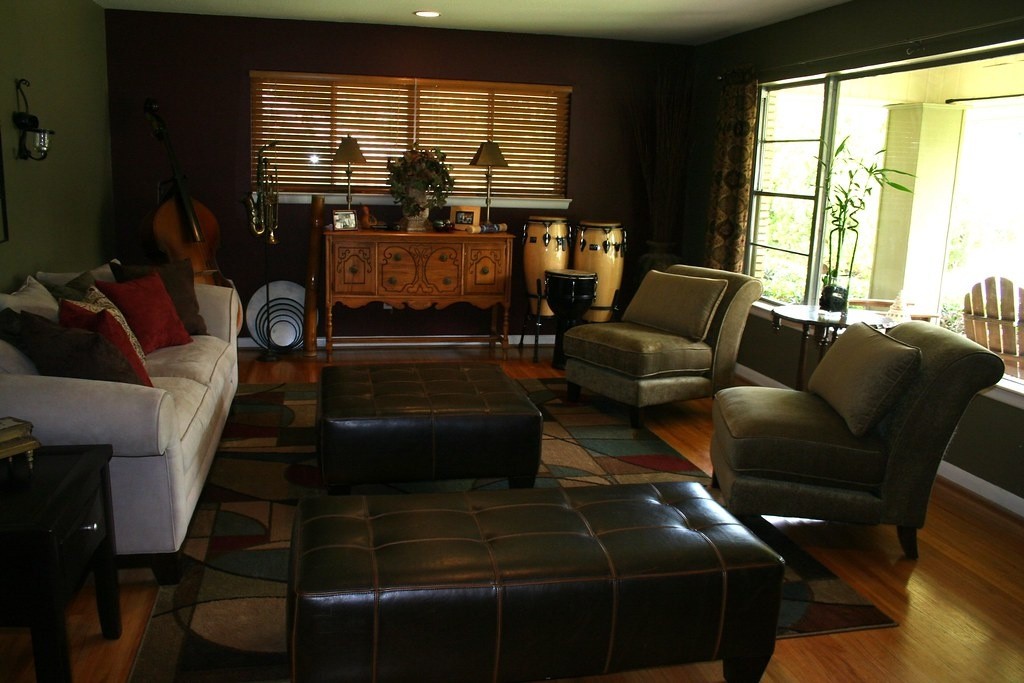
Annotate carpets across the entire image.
[129,374,899,683]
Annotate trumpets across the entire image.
[264,157,280,245]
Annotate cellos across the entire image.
[143,96,246,340]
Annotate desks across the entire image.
[322,226,515,364]
[0,443,123,683]
[771,304,912,392]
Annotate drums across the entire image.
[572,219,626,322]
[544,268,598,370]
[522,216,570,317]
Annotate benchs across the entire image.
[286,481,786,683]
[315,364,543,497]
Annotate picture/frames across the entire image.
[450,205,480,230]
[332,209,358,231]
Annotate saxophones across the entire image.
[239,139,281,245]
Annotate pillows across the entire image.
[0,257,212,388]
[808,321,921,438]
[622,270,729,341]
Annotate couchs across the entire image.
[709,319,1005,559]
[562,264,763,429]
[0,264,244,584]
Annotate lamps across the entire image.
[469,142,509,226]
[330,134,366,210]
[13,79,56,161]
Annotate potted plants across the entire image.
[808,136,921,317]
[385,141,456,230]
